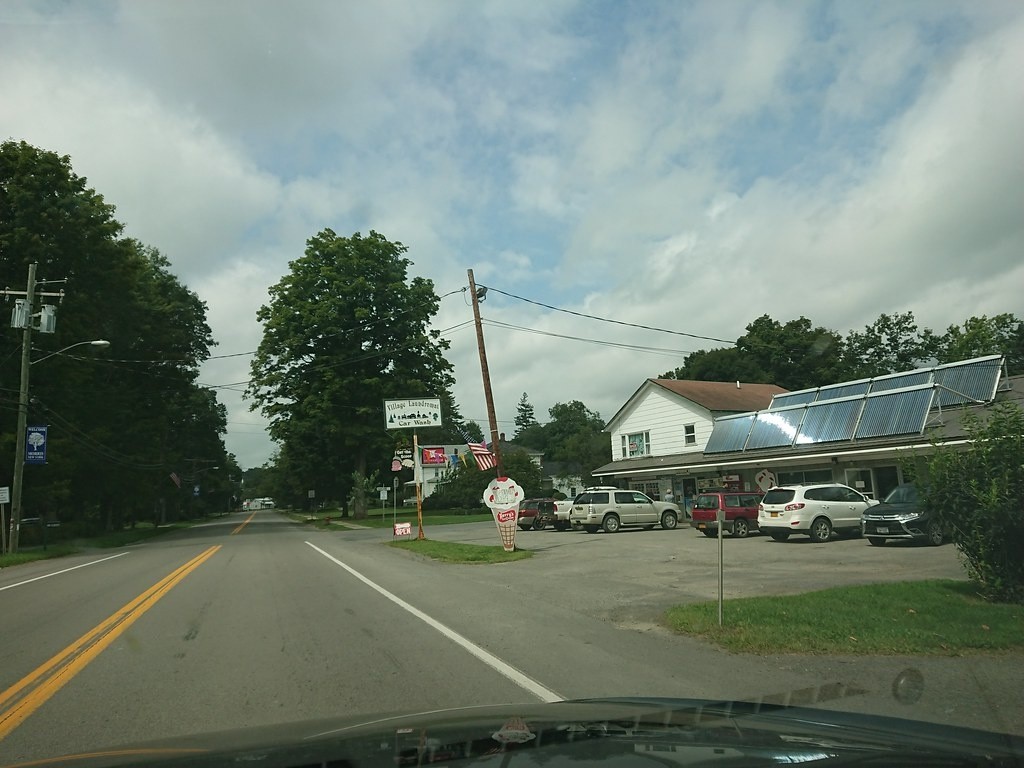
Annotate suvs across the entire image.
[570,488,682,534]
[516,498,558,531]
[689,489,770,538]
[757,482,880,543]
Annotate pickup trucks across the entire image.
[537,496,586,531]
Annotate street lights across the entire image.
[189,466,219,522]
[9,339,110,552]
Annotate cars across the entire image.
[861,484,953,548]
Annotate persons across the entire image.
[664,489,675,502]
[646,488,654,502]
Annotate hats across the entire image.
[667,489,671,493]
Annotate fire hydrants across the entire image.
[325,516,330,524]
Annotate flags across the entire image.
[460,430,497,470]
